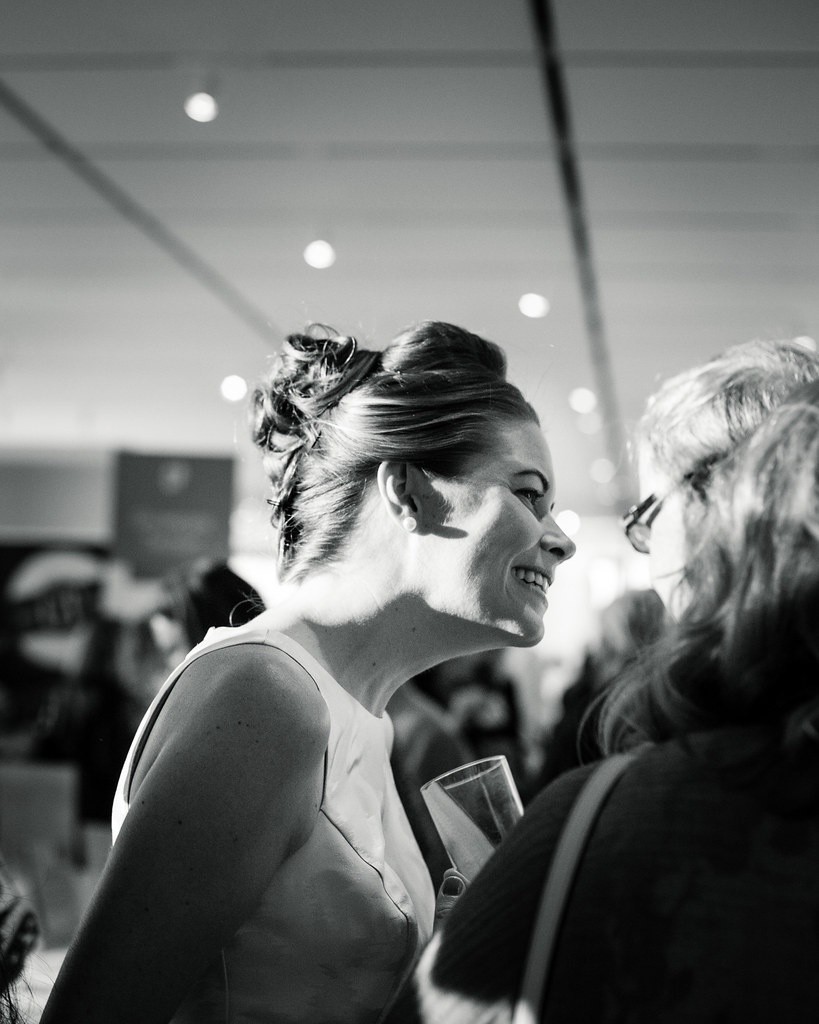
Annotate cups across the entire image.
[420,755,525,890]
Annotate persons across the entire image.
[416,330,819,1024]
[36,322,574,1024]
[0,551,676,899]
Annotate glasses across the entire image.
[620,451,729,555]
